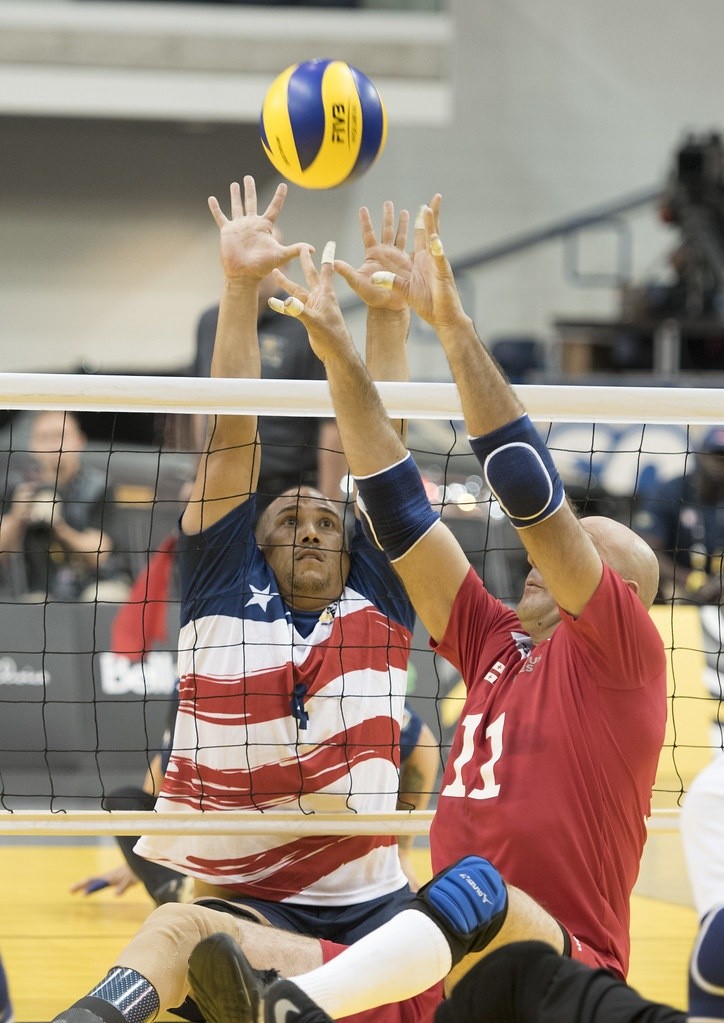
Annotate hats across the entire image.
[702,427,724,455]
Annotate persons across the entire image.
[430,749,724,1023]
[65,680,440,906]
[0,411,135,603]
[191,227,349,511]
[52,194,668,1023]
[132,178,417,948]
[634,424,724,606]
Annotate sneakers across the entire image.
[187,933,333,1023]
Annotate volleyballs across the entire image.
[258,56,391,194]
